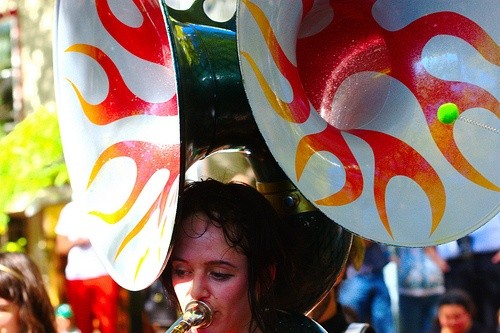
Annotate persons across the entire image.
[0,250,58,333]
[158,178,500,333]
[56,201,129,333]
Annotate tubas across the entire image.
[166,300,215,333]
